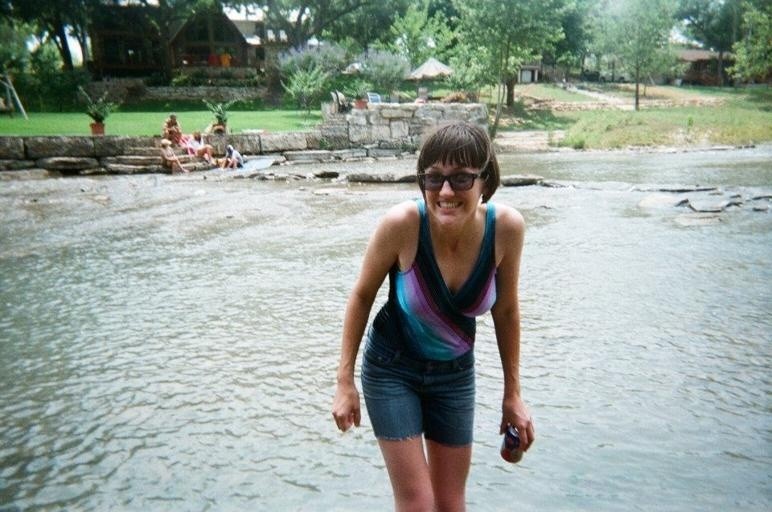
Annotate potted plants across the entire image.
[202,96,242,131]
[78,85,126,135]
[342,78,372,111]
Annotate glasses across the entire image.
[418,170,481,191]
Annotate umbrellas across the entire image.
[406,58,453,84]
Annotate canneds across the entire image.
[500,425,523,463]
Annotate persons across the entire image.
[158,138,192,175]
[161,114,183,145]
[349,94,372,110]
[187,131,213,164]
[332,121,535,511]
[169,131,196,156]
[413,87,430,104]
[222,145,245,170]
